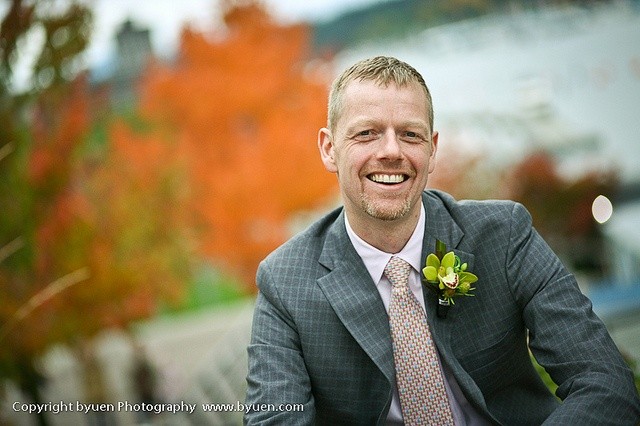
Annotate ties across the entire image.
[384,255,456,426]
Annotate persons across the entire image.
[244,54,636,426]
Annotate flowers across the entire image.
[421,238,477,318]
